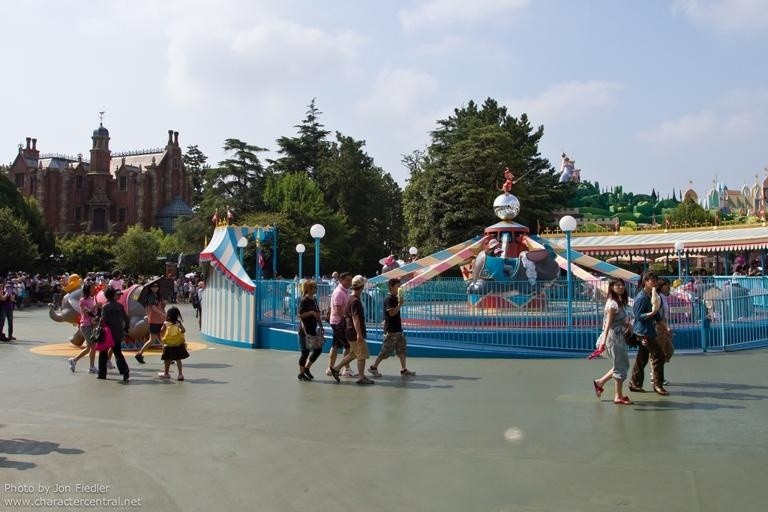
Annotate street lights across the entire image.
[558,214,578,327]
[237,237,248,265]
[49,251,65,280]
[675,240,685,279]
[310,224,327,284]
[296,243,306,279]
[408,245,418,263]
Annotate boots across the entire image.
[0,334,9,341]
[8,335,17,340]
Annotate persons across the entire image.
[649,278,671,387]
[297,280,325,381]
[330,273,375,384]
[493,248,513,276]
[325,271,357,377]
[0,270,205,382]
[593,278,633,405]
[600,206,765,232]
[367,278,416,377]
[486,238,502,257]
[258,252,418,323]
[628,271,669,394]
[634,258,768,297]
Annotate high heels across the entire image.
[123,372,129,380]
[653,384,669,395]
[628,382,648,393]
[97,374,106,380]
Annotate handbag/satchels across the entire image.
[625,331,639,348]
[95,328,115,349]
[90,327,105,343]
[306,333,326,349]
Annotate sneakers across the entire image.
[88,367,98,374]
[164,360,175,364]
[650,377,670,386]
[177,373,185,380]
[298,373,312,382]
[107,362,116,368]
[327,365,416,384]
[68,358,76,372]
[304,367,314,378]
[134,353,146,363]
[158,372,171,379]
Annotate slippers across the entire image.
[614,396,634,405]
[593,380,604,398]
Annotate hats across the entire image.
[656,279,670,292]
[351,275,367,289]
[116,289,124,295]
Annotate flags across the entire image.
[227,210,235,219]
[211,212,218,223]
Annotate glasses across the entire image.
[150,284,160,288]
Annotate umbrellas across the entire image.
[606,254,652,270]
[654,252,707,263]
